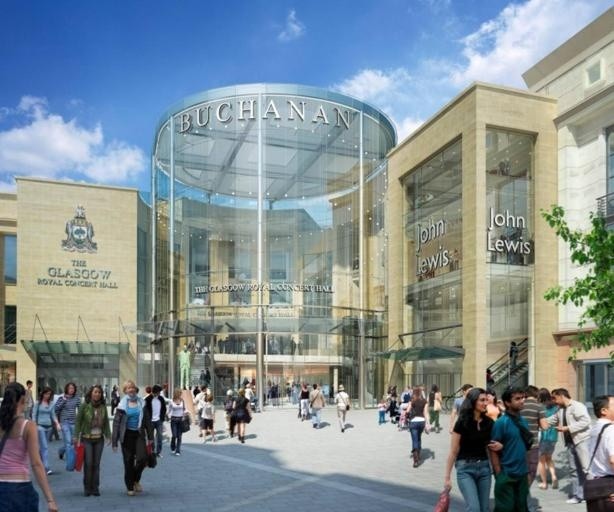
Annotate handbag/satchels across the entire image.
[181,419,190,433]
[435,489,451,512]
[64,441,85,472]
[519,425,536,452]
[346,405,350,410]
[583,477,614,501]
[146,441,157,468]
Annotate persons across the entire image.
[452,248,459,269]
[508,341,519,369]
[265,379,352,432]
[489,228,529,265]
[374,379,614,512]
[487,367,495,388]
[417,266,435,281]
[0,370,262,512]
[448,251,454,271]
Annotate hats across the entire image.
[338,384,345,391]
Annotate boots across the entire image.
[412,448,422,467]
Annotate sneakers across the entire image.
[133,481,143,492]
[538,482,547,490]
[551,479,559,488]
[46,469,52,476]
[171,449,180,456]
[238,433,245,444]
[567,497,586,504]
[84,489,100,497]
[58,448,64,459]
[157,453,164,458]
[127,485,135,496]
